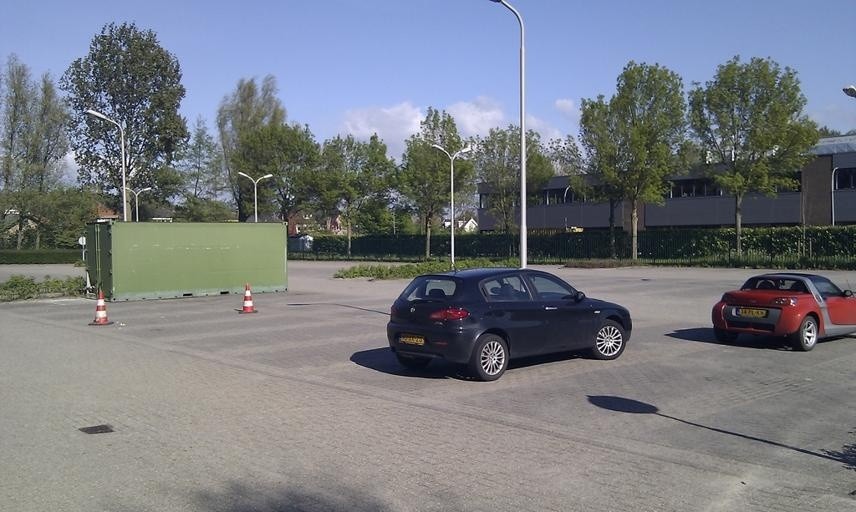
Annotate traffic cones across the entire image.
[88,290,114,325]
[239,282,258,314]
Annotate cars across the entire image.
[387,268,632,382]
[712,273,856,352]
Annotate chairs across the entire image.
[791,281,807,292]
[501,284,513,297]
[758,281,775,290]
[429,289,445,297]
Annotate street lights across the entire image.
[432,144,472,271]
[85,109,150,222]
[237,171,273,222]
[491,0,527,269]
